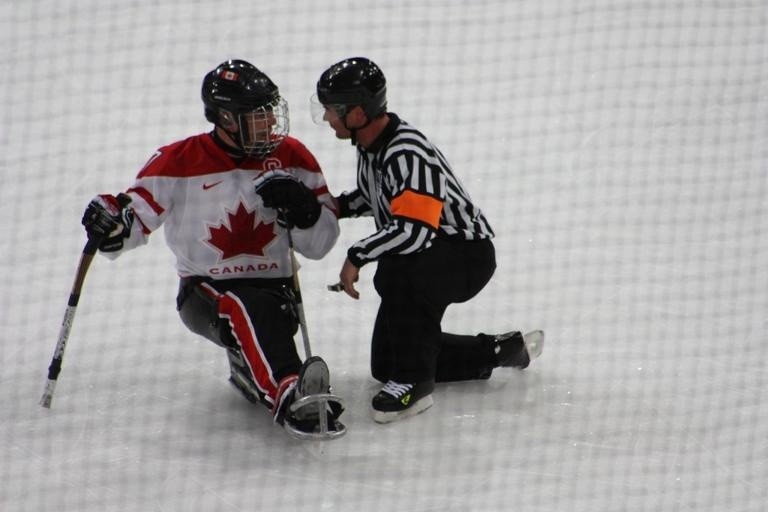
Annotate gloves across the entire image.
[83,190,136,254]
[252,169,323,232]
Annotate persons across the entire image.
[80,59,340,426]
[313,57,532,412]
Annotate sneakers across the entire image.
[284,355,333,436]
[371,370,436,413]
[495,329,531,368]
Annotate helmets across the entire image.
[317,56,387,119]
[200,58,280,152]
